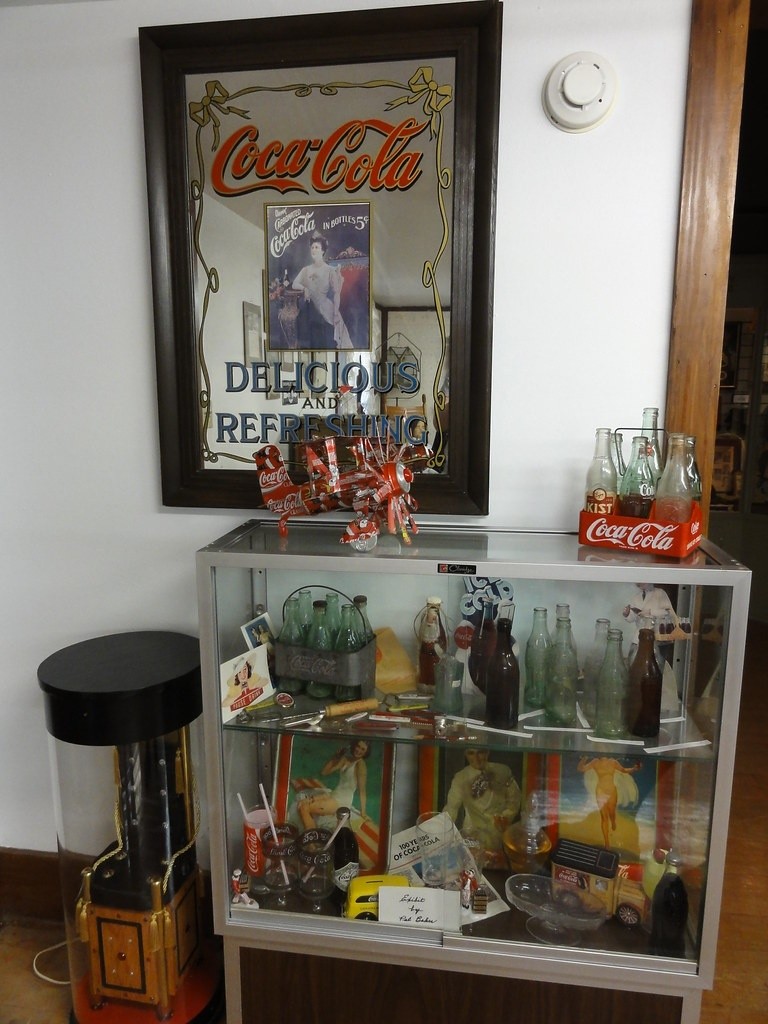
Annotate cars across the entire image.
[339,873,410,923]
[552,845,647,928]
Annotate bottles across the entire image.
[273,586,371,704]
[587,429,617,515]
[334,808,361,903]
[414,600,577,730]
[618,436,654,517]
[649,855,688,955]
[656,433,689,523]
[643,408,662,486]
[685,437,702,500]
[585,613,692,738]
[504,814,551,871]
[610,434,626,493]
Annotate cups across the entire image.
[240,806,278,895]
[455,826,484,907]
[416,813,454,885]
[296,827,334,901]
[264,824,299,898]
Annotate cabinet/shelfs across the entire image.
[193,518,751,1024]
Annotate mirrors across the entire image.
[137,0,504,517]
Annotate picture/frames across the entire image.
[264,200,372,352]
[244,301,327,404]
